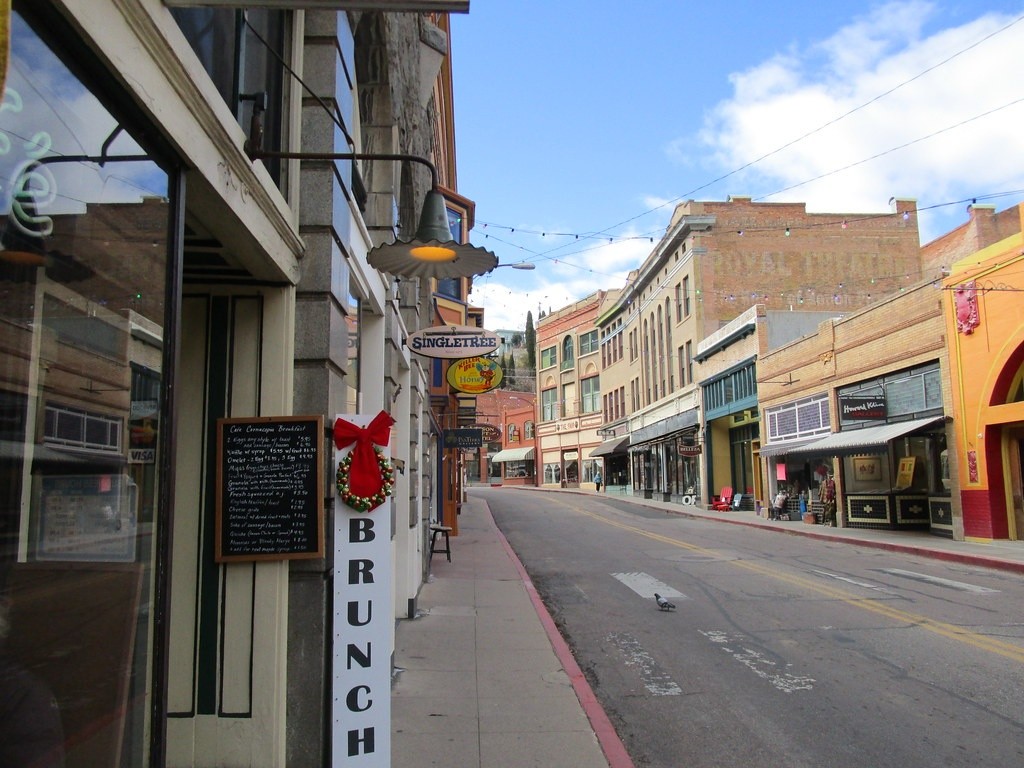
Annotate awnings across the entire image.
[759,432,830,458]
[588,435,629,458]
[786,414,952,459]
[492,446,535,463]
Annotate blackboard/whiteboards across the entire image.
[214,414,325,563]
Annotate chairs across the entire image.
[729,494,742,512]
[712,487,733,510]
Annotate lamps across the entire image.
[244,139,499,281]
[473,263,535,280]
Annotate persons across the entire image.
[594,471,603,493]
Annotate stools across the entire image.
[430,526,452,563]
[717,505,729,512]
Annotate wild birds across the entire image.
[654,593,676,611]
